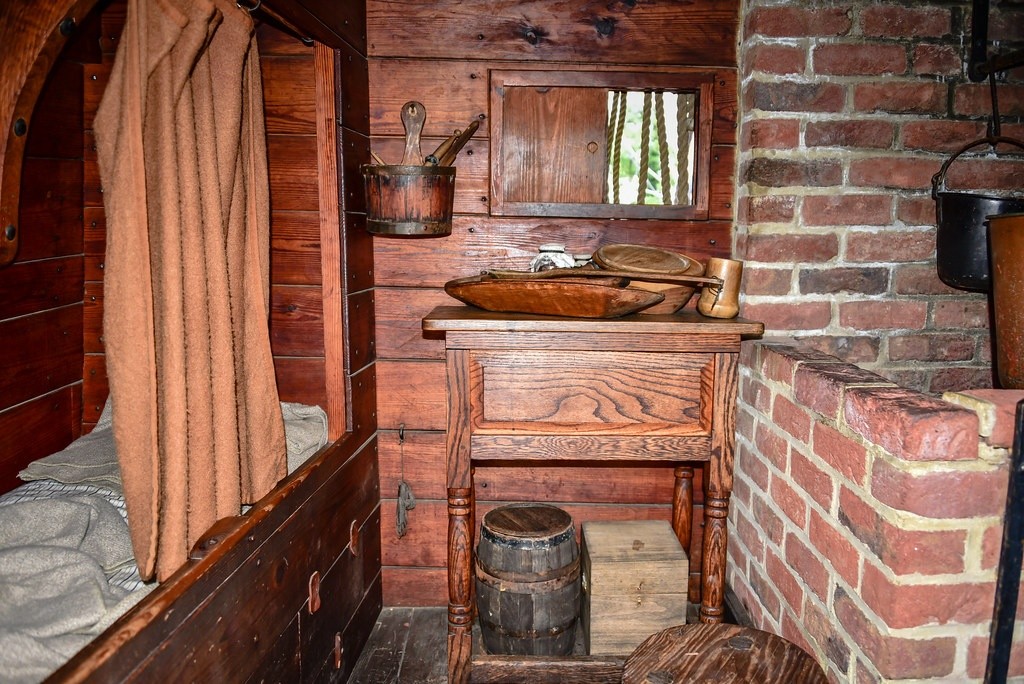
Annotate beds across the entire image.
[0,0,387,684]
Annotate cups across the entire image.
[698,258,743,319]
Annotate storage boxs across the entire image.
[576,518,692,656]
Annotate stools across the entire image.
[621,618,833,684]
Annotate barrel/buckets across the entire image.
[932,136,1024,291]
[474,503,582,657]
[361,163,457,236]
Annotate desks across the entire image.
[421,302,769,684]
